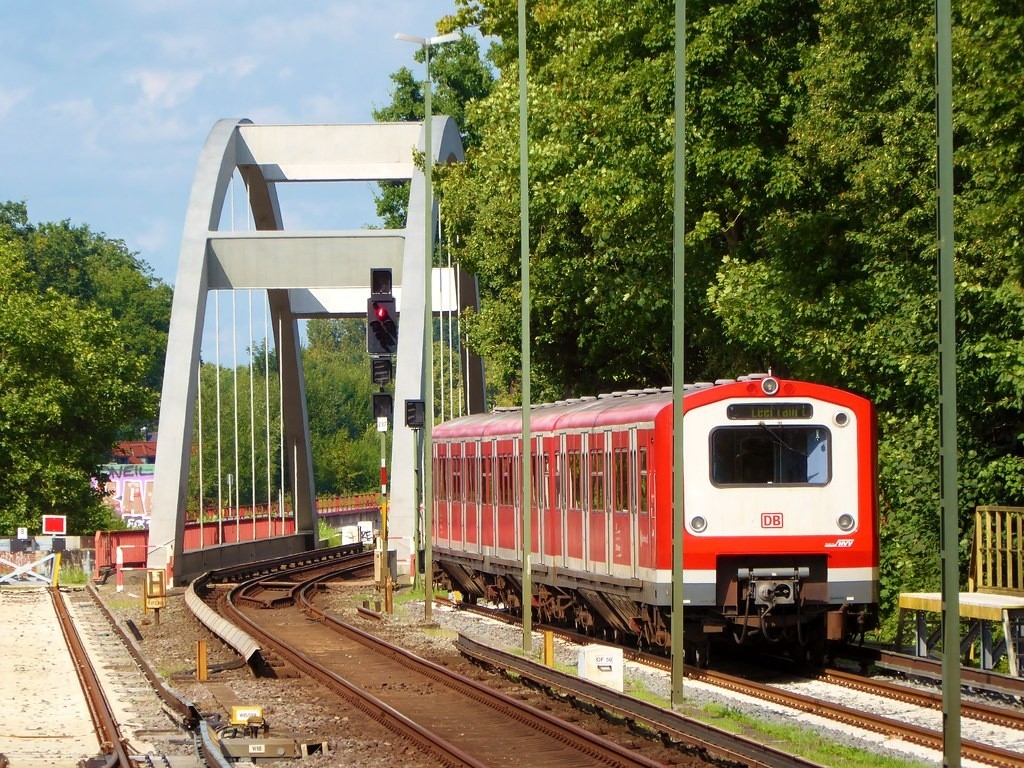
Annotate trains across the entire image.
[417,365,881,674]
